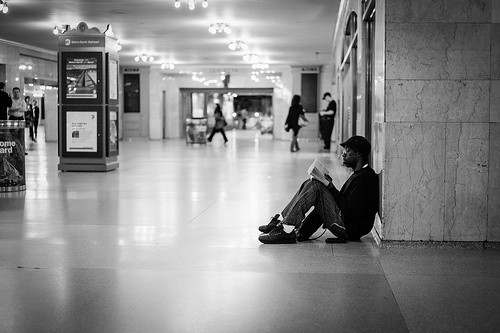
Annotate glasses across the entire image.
[342,148,357,155]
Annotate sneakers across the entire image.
[258,225,297,244]
[259,214,281,233]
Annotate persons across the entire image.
[257,134,378,245]
[285,94,309,151]
[319,91,337,151]
[0,81,39,155]
[234,106,248,129]
[207,102,228,143]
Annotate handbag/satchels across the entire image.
[298,115,309,127]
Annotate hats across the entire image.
[340,136,371,157]
[323,93,331,100]
